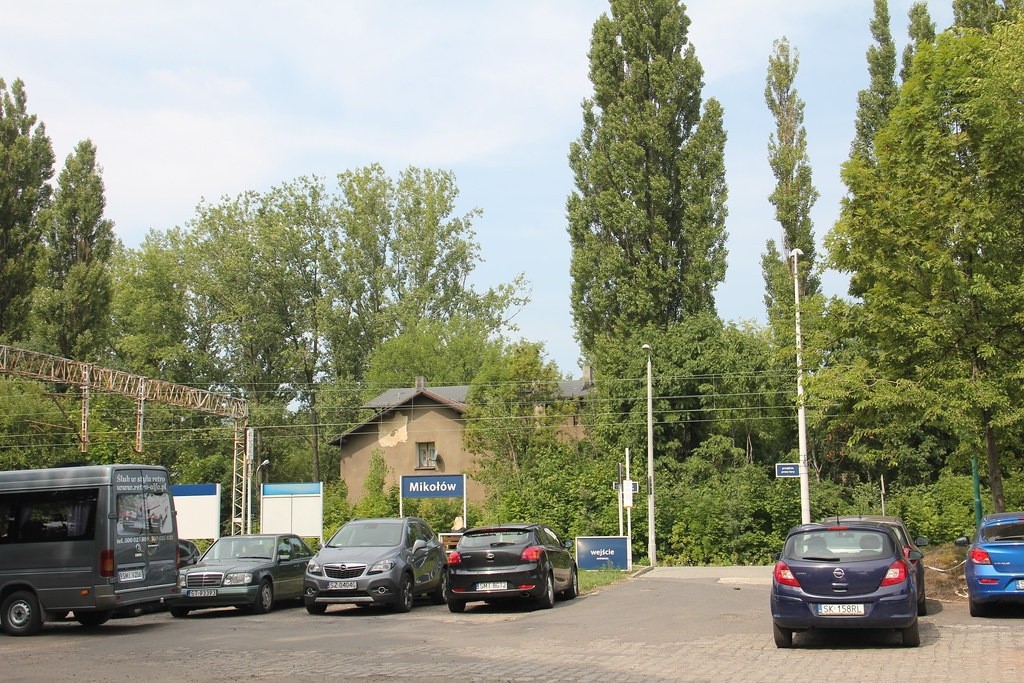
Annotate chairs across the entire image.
[803,537,832,555]
[856,534,881,557]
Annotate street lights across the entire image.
[788,246,813,523]
[255,459,270,489]
[640,342,658,563]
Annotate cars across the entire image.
[169,533,316,616]
[303,516,448,615]
[954,511,1024,616]
[769,514,929,648]
[178,538,201,568]
[446,523,579,612]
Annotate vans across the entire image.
[0,463,183,635]
